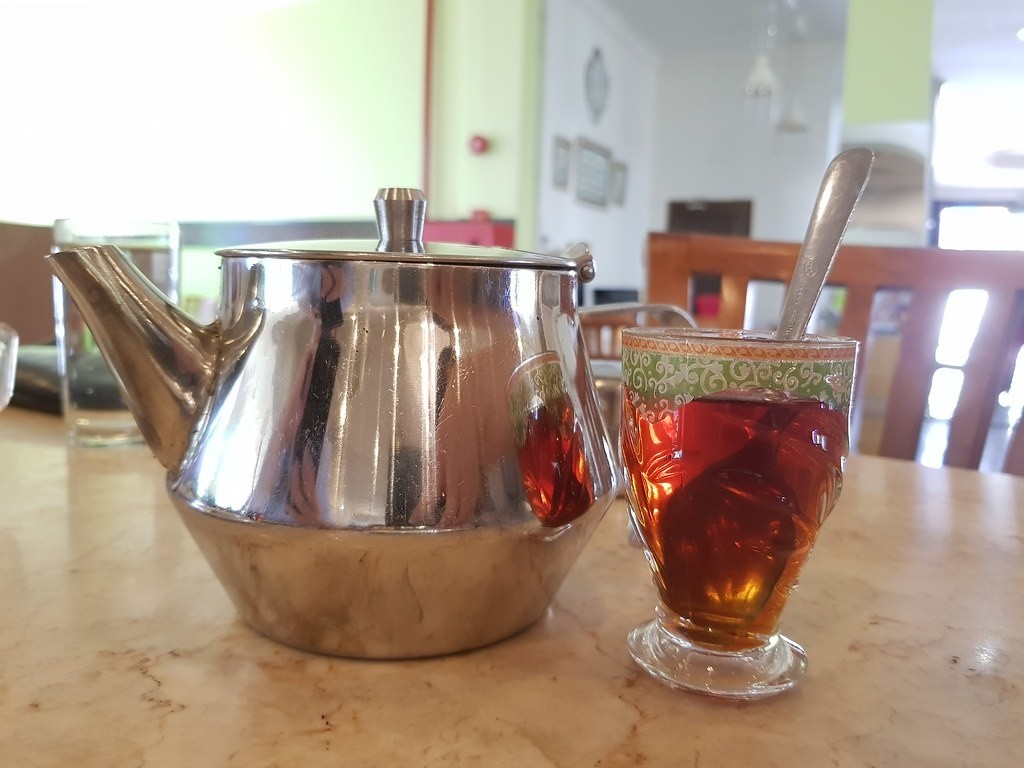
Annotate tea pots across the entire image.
[48,188,701,660]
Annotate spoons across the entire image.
[775,147,874,339]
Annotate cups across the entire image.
[53,215,179,448]
[620,322,861,699]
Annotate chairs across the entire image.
[640,226,1024,471]
